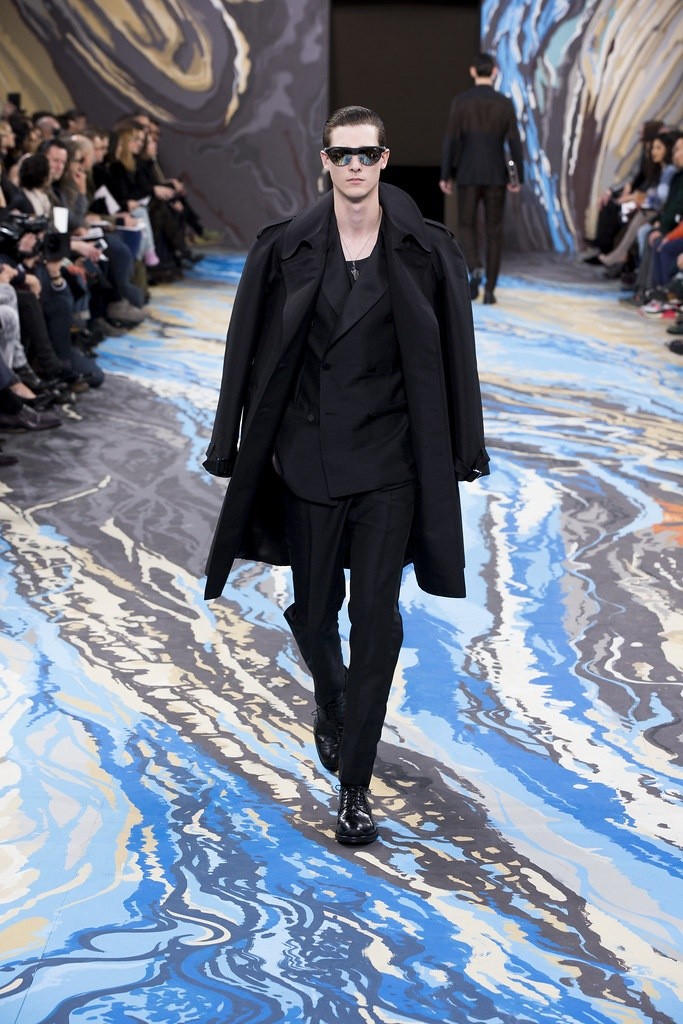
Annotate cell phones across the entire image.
[94,239,108,251]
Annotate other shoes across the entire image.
[196,228,225,244]
[80,299,146,355]
[0,366,101,429]
[153,248,206,282]
[484,292,495,303]
[583,253,609,267]
[0,451,19,465]
[469,270,481,299]
[622,286,683,355]
[583,236,605,250]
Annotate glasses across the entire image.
[73,157,85,164]
[324,146,386,166]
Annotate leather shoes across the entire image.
[311,697,346,772]
[334,785,378,843]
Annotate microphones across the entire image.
[21,219,48,233]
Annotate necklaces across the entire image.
[341,229,374,282]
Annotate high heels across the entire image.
[600,251,626,271]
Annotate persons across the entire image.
[0,92,207,467]
[202,106,491,847]
[439,54,524,304]
[582,120,683,356]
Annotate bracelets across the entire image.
[51,275,61,281]
